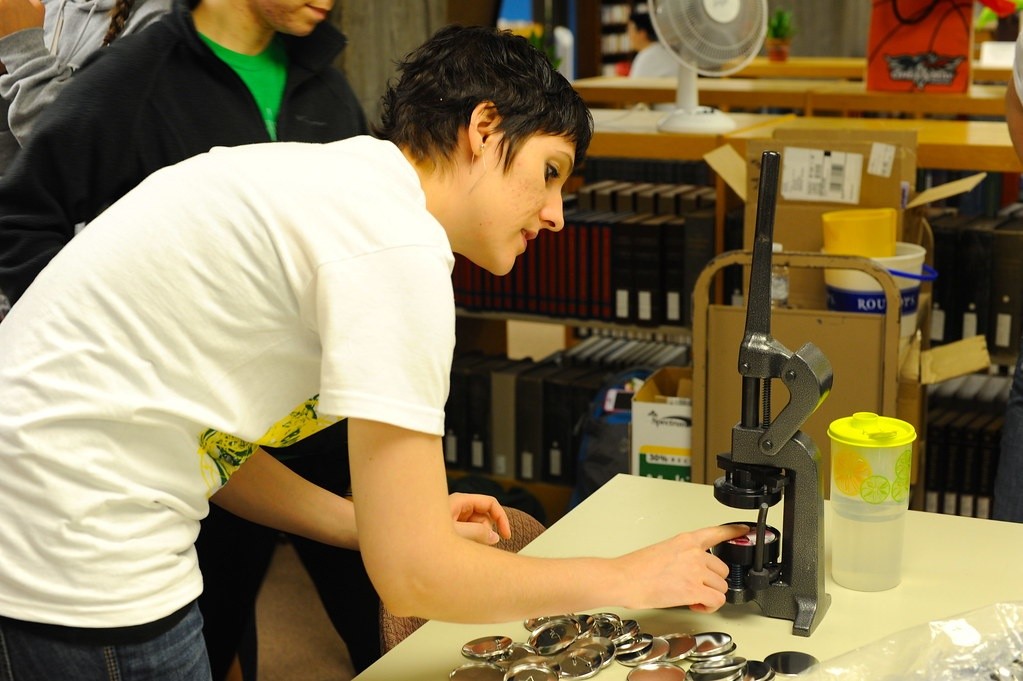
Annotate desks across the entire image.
[348,473,1023,681]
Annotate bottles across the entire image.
[826,411,918,592]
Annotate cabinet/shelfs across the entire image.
[574,75,1009,122]
[719,57,1013,86]
[440,107,1023,523]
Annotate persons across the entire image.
[1,0,406,681]
[2,21,759,676]
[1,0,175,189]
[626,13,680,78]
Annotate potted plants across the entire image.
[764,8,798,62]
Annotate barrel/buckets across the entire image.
[821,208,896,258]
[820,241,938,337]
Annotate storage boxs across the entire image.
[632,365,693,483]
[700,119,987,311]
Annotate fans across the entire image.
[647,0,768,133]
